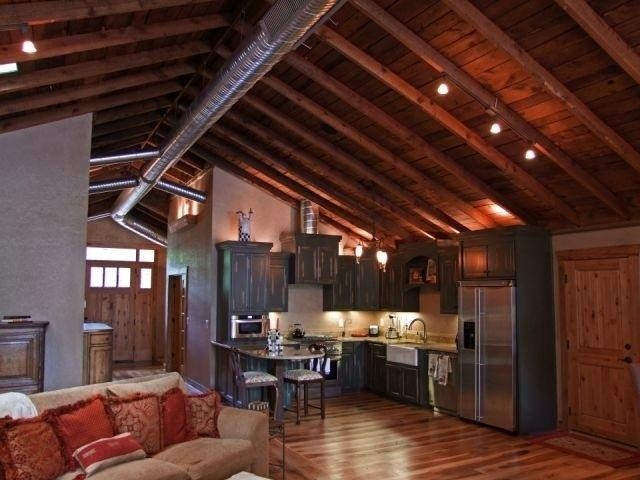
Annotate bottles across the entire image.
[278,335,284,352]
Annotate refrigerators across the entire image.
[453,279,519,436]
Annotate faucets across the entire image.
[408,318,428,341]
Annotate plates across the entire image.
[350,334,367,337]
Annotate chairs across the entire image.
[229,349,280,420]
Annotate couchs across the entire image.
[0,370,269,479]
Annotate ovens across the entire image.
[425,349,460,418]
[299,353,342,400]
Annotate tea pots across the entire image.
[290,323,305,338]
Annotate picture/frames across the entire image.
[426,258,435,284]
[409,267,425,286]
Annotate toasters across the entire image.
[369,325,379,337]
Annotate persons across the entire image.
[410,269,421,281]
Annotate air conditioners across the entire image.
[168,214,198,233]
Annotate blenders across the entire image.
[384,313,399,340]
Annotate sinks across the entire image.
[392,342,431,347]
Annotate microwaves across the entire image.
[230,316,270,338]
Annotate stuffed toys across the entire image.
[235,204,255,242]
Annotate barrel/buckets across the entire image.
[300,199,318,234]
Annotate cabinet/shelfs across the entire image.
[366,341,386,397]
[284,344,328,425]
[436,246,458,315]
[0,320,50,392]
[84,322,114,385]
[270,252,291,313]
[385,364,419,404]
[279,233,342,285]
[400,239,439,295]
[355,253,420,311]
[458,227,557,280]
[322,255,355,311]
[339,343,372,390]
[216,239,273,315]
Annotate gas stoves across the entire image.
[288,336,343,353]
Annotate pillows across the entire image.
[103,374,182,401]
[160,387,186,445]
[0,418,66,478]
[106,387,164,454]
[180,391,221,439]
[41,394,121,472]
[71,431,145,478]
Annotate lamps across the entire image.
[355,180,388,272]
[438,72,536,160]
[17,21,38,55]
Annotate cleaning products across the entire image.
[415,332,420,343]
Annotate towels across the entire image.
[437,355,454,387]
[427,353,439,379]
[308,356,331,375]
